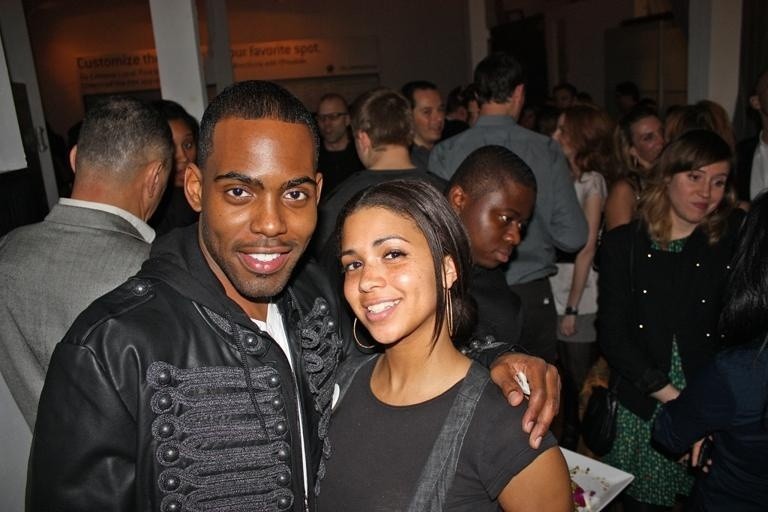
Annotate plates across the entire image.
[558,445,634,511]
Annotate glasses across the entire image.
[314,111,349,123]
